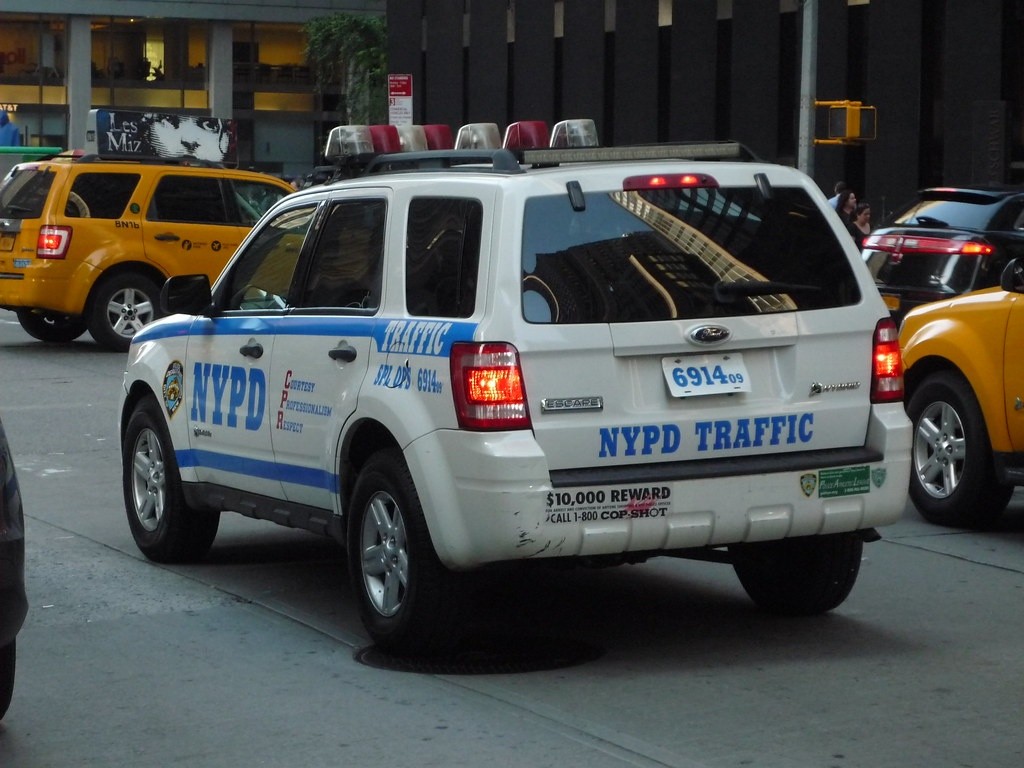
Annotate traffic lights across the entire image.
[858,106,879,141]
[827,105,859,141]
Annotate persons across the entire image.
[835,190,856,229]
[828,182,847,212]
[0,111,21,146]
[847,203,870,253]
[249,170,300,215]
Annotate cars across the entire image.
[855,187,1024,334]
[895,256,1024,528]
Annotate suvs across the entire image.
[116,117,916,665]
[1,147,385,355]
